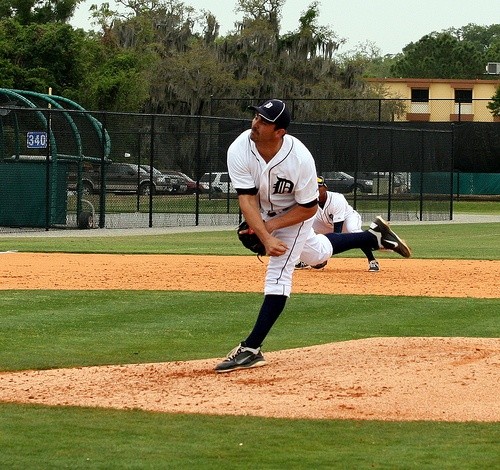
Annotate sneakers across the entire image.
[295,261,311,270]
[368,215,412,259]
[367,259,380,272]
[214,341,269,372]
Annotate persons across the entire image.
[295,176,380,272]
[214,98,411,373]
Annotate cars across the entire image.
[68,163,373,196]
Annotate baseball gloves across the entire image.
[238,219,269,257]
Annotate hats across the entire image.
[315,176,328,188]
[247,98,291,129]
